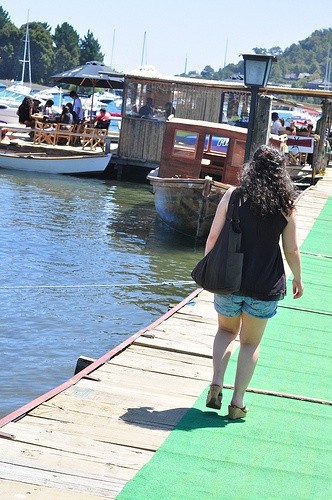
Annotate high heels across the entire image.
[206,385,222,410]
[228,405,247,419]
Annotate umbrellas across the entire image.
[48,60,124,124]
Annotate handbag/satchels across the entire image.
[191,187,244,293]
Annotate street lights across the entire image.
[239,53,278,163]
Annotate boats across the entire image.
[0,148,113,174]
[41,28,148,152]
[147,115,288,245]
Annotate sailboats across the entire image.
[0,7,53,108]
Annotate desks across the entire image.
[287,135,315,166]
[82,127,106,153]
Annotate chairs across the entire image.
[33,115,111,146]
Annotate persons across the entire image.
[17,96,54,127]
[139,98,153,115]
[205,146,304,420]
[279,118,286,128]
[289,122,296,136]
[94,109,110,130]
[164,102,175,117]
[271,112,283,134]
[69,91,84,124]
[62,103,78,124]
